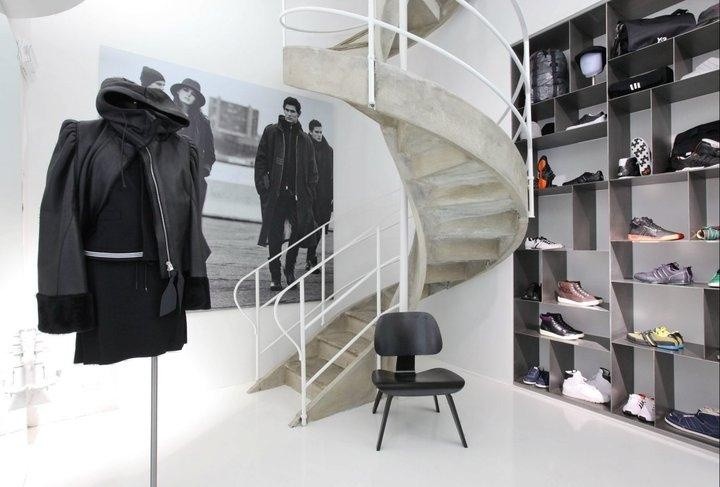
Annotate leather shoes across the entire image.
[306,257,320,273]
[284,268,295,288]
[269,282,281,289]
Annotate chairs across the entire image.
[371,309,470,451]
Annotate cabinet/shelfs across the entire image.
[505,0,720,460]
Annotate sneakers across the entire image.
[535,368,549,388]
[540,313,584,340]
[565,110,606,132]
[523,366,543,384]
[520,283,541,301]
[708,268,720,287]
[637,397,655,423]
[537,155,556,190]
[563,170,604,185]
[524,237,564,250]
[665,408,720,442]
[557,280,603,306]
[622,393,646,417]
[631,137,653,176]
[627,328,684,351]
[627,216,684,241]
[617,157,641,179]
[697,226,719,240]
[675,139,719,172]
[635,264,693,285]
[562,368,610,404]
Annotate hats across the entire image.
[140,66,165,88]
[170,79,206,107]
[575,46,606,67]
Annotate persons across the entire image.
[169,77,217,263]
[305,119,333,275]
[138,66,165,93]
[252,96,320,291]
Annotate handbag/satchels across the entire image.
[529,48,567,104]
[668,121,720,171]
[698,4,720,27]
[608,65,673,99]
[611,9,696,59]
